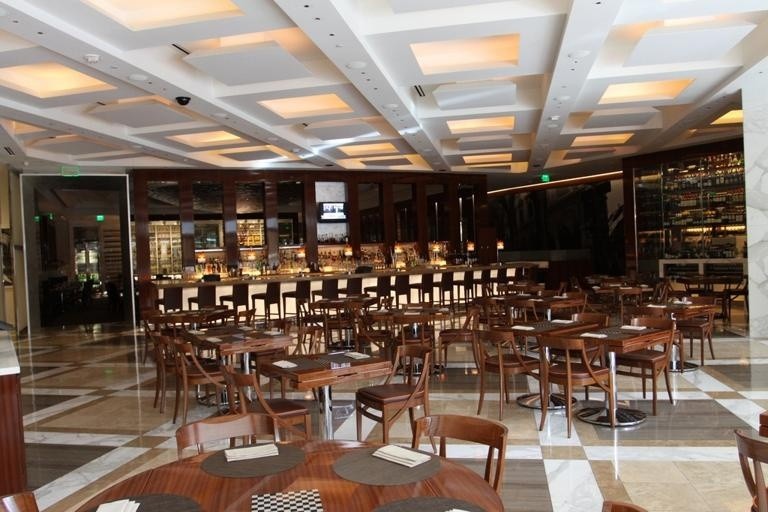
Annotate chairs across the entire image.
[603,497,648,512]
[1,493,39,512]
[144,264,746,455]
[730,427,767,512]
[412,414,506,496]
[176,412,278,465]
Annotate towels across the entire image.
[223,444,279,462]
[94,498,140,511]
[371,445,429,468]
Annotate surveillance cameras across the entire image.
[176,97,191,105]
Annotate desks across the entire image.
[758,409,766,438]
[67,442,503,511]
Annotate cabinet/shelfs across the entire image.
[131,219,184,275]
[623,138,745,279]
[236,218,266,250]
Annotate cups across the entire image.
[319,231,344,243]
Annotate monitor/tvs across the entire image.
[318,202,350,223]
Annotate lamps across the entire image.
[192,240,510,272]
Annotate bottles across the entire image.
[664,151,745,223]
[203,256,224,275]
